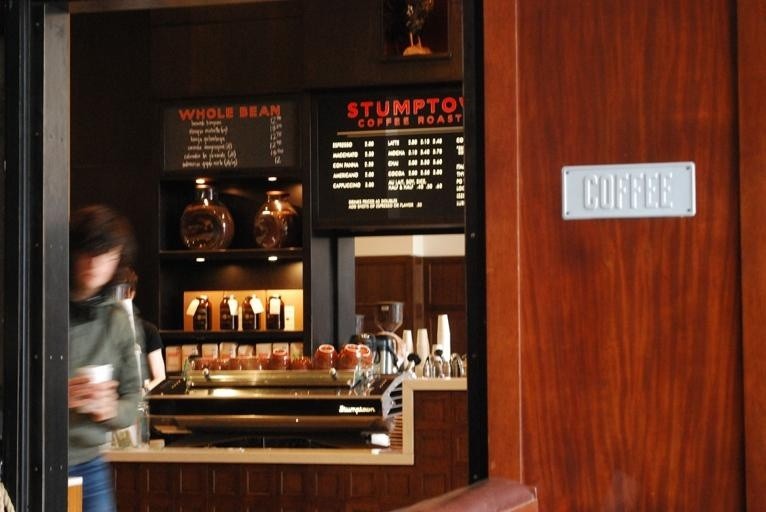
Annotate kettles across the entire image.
[422,351,450,378]
[375,336,398,374]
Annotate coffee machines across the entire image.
[375,301,406,374]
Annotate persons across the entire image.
[67,202,143,511]
[113,264,165,390]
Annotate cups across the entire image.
[402,313,451,368]
[79,362,118,423]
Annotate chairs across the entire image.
[387,475,538,512]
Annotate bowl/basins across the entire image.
[193,343,372,370]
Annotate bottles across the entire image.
[135,401,151,449]
[178,177,234,249]
[193,294,285,330]
[252,178,303,249]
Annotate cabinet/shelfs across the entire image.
[155,168,338,377]
